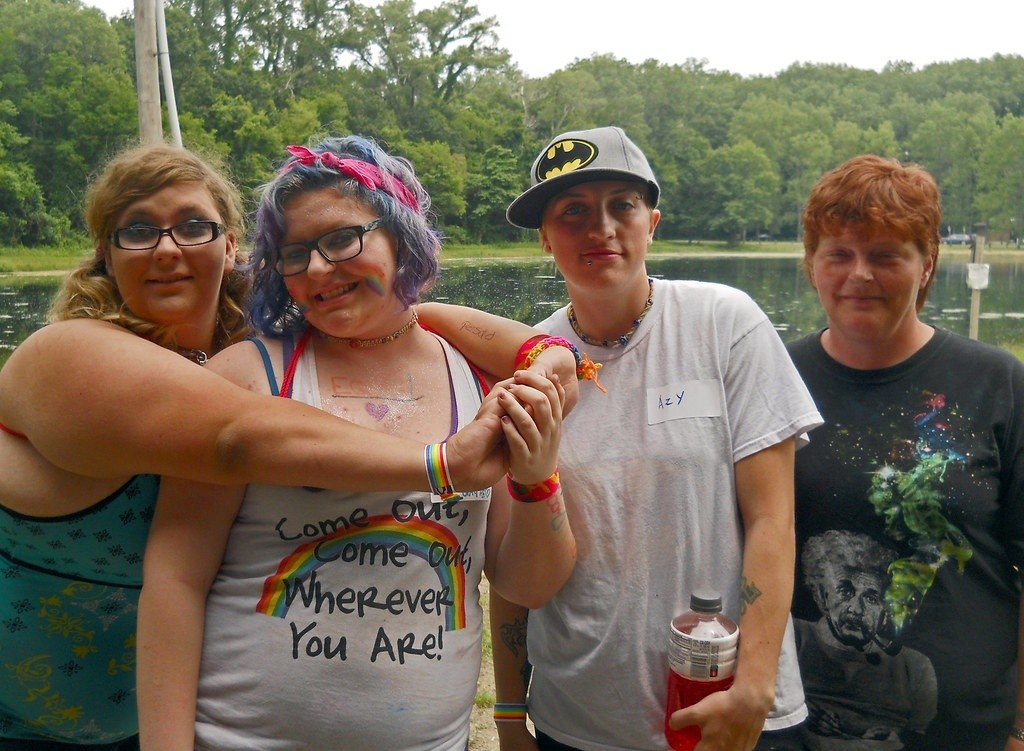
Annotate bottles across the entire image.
[664,590,740,750]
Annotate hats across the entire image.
[505,127,661,230]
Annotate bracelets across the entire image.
[1010,725,1024,740]
[512,333,607,393]
[506,466,559,503]
[425,440,455,501]
[493,702,529,723]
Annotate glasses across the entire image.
[108,221,227,251]
[270,216,392,277]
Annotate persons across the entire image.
[757,151,1024,751]
[136,136,578,751]
[1,133,581,751]
[488,126,815,751]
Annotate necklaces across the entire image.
[162,340,210,367]
[567,277,653,349]
[302,303,417,347]
